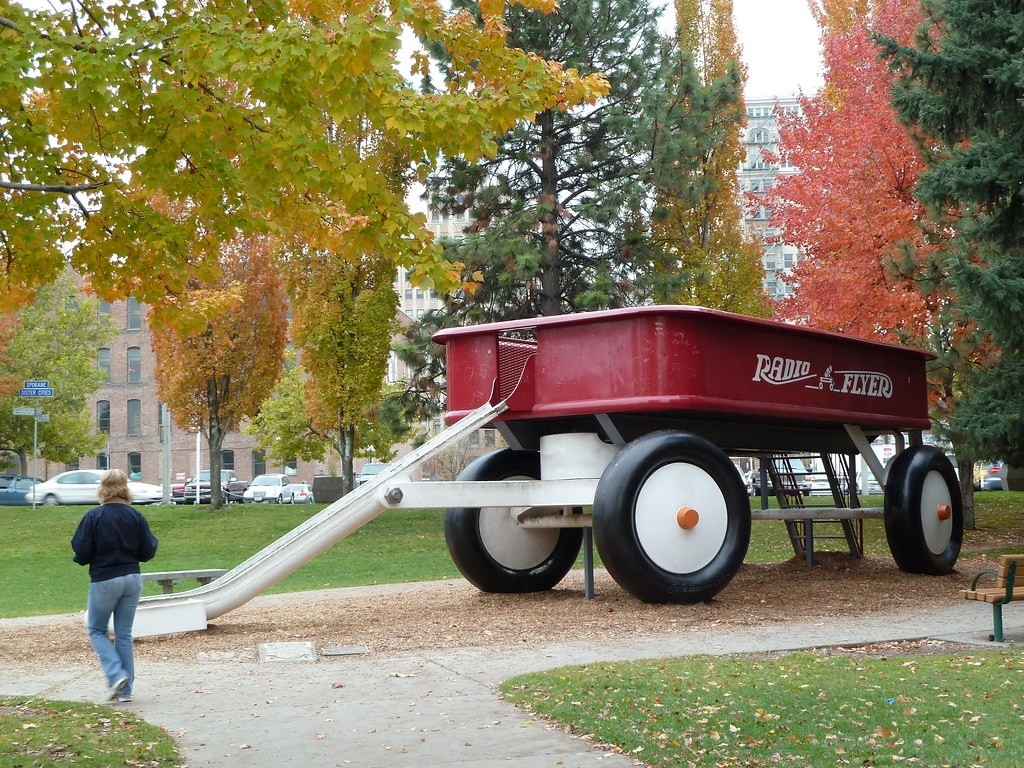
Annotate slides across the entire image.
[84,352,536,638]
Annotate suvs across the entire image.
[353,463,391,486]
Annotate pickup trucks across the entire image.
[184,468,248,504]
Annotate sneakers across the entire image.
[105,676,133,702]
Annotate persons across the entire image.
[70,467,160,703]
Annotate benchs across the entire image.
[960,554,1024,642]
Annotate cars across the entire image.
[24,470,163,505]
[293,483,313,505]
[734,443,1004,496]
[243,472,294,504]
[1,474,45,505]
